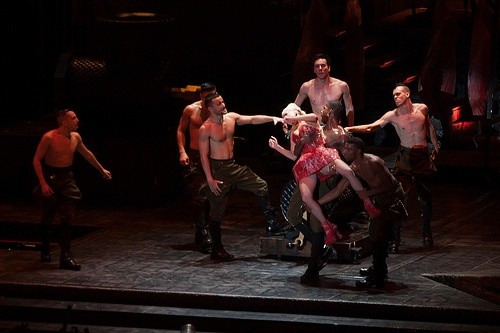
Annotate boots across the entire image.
[60,225,81,269]
[41,224,51,263]
[356,241,390,288]
[209,219,235,261]
[295,220,333,270]
[257,196,287,233]
[302,231,325,281]
[193,212,213,245]
[423,203,432,248]
[359,240,388,274]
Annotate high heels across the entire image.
[364,199,381,218]
[322,221,343,245]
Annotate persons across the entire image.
[176,55,438,290]
[33,110,111,272]
